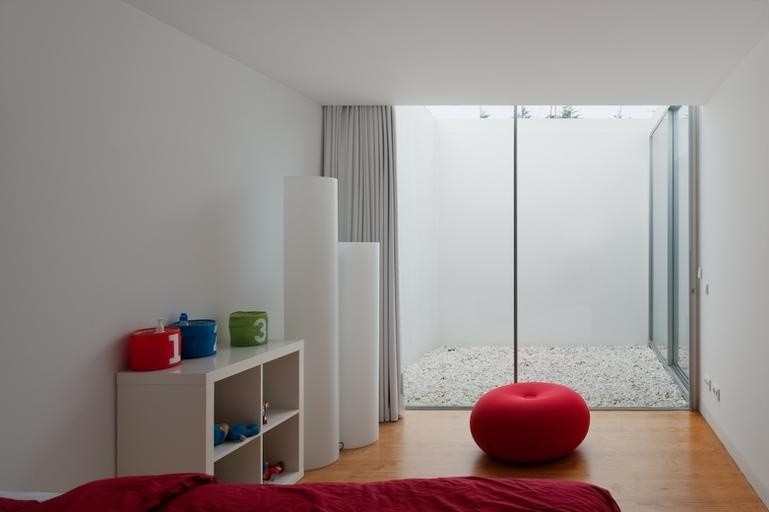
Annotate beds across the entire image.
[0,466,624,512]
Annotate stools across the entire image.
[467,381,594,466]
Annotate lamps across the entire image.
[335,235,384,454]
[276,172,344,473]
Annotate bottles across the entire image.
[154,316,168,334]
[177,313,191,327]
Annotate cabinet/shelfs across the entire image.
[114,336,309,486]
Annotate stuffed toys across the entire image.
[215,420,260,444]
